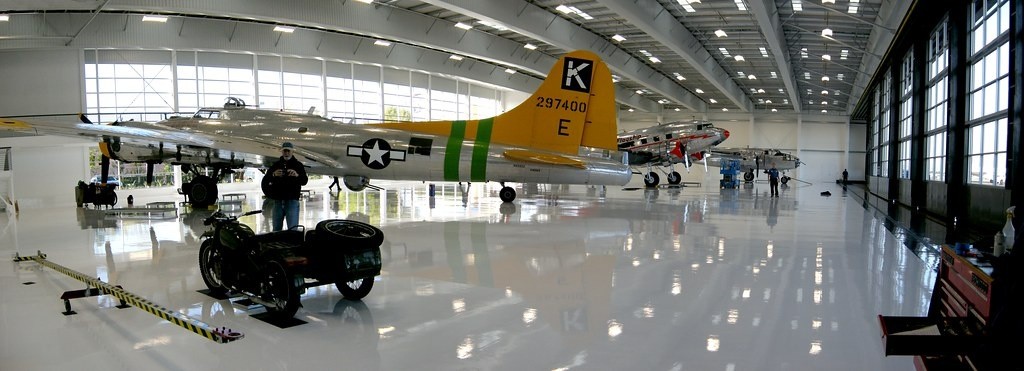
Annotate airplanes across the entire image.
[690,148,813,186]
[616,117,730,189]
[1,50,632,204]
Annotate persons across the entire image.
[329,176,342,190]
[459,181,471,185]
[843,169,848,186]
[267,142,308,231]
[768,163,779,198]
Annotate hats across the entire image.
[281,142,293,150]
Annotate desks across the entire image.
[222,194,246,201]
[219,200,243,213]
[146,202,175,217]
[301,189,315,197]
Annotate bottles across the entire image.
[993,231,1006,257]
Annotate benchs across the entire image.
[111,208,178,220]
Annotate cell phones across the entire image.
[280,170,290,177]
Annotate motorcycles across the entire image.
[198,209,384,327]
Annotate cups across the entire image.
[955,242,970,257]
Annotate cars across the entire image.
[90,175,119,187]
